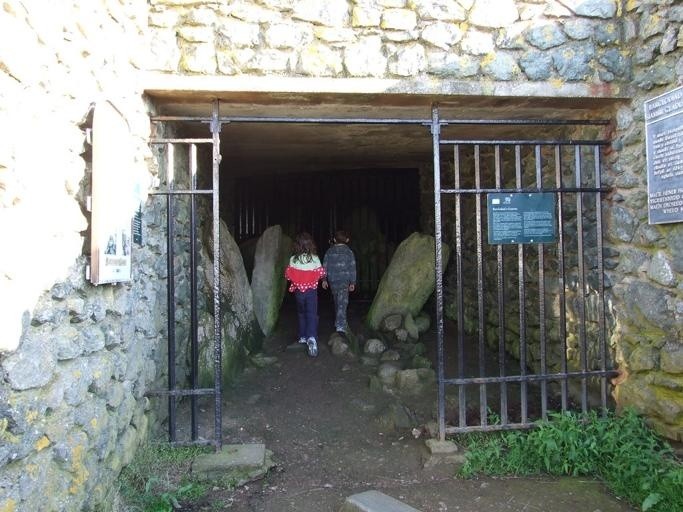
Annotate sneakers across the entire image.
[306,336,317,357]
[298,336,306,344]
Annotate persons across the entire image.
[284,232,326,357]
[321,231,357,337]
